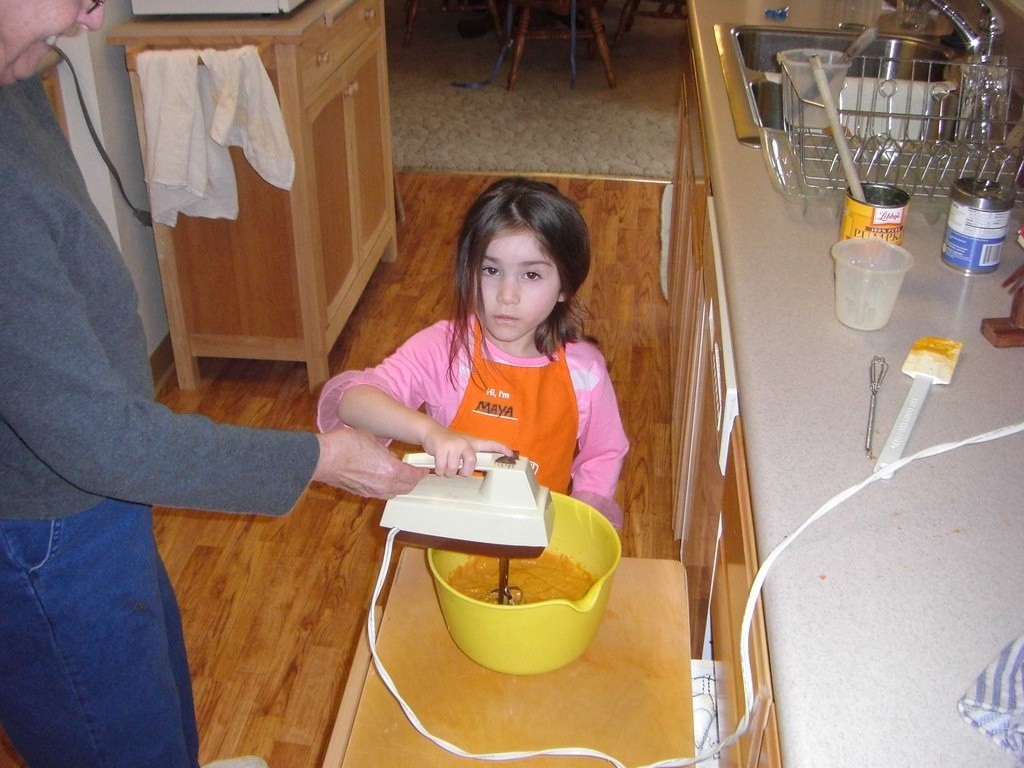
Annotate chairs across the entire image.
[403,0,686,90]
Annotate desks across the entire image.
[323,545,696,768]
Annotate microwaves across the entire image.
[130,0,308,16]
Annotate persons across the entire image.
[317,176,629,526]
[0,0,432,768]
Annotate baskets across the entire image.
[776,53,1024,197]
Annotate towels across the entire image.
[201,45,295,192]
[137,44,239,228]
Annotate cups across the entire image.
[894,0,928,31]
[961,55,1009,146]
[833,238,913,331]
[781,47,852,129]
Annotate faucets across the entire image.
[930,0,1006,58]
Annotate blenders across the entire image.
[380,451,555,608]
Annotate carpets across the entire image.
[385,0,687,180]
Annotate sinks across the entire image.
[712,23,966,75]
[723,75,1024,149]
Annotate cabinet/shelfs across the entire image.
[670,55,710,538]
[124,0,398,397]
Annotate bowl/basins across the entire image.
[427,486,622,675]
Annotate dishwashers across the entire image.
[681,197,738,660]
